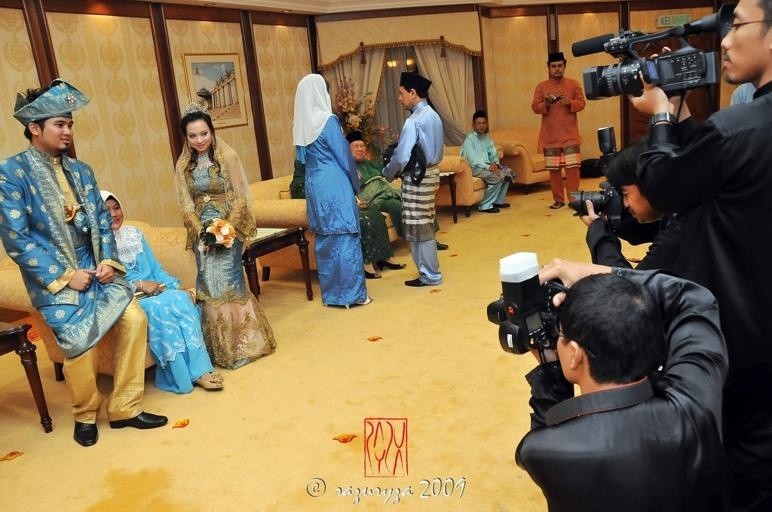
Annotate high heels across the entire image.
[325,296,373,309]
[364,259,407,278]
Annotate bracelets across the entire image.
[137,278,143,293]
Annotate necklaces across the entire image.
[197,151,208,161]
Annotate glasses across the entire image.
[724,20,768,34]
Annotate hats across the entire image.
[549,52,564,61]
[346,131,361,143]
[400,72,431,91]
[473,111,486,121]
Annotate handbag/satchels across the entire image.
[384,143,425,185]
[291,160,305,198]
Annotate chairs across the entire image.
[243,173,404,282]
[489,126,569,194]
[434,144,504,215]
[0,218,204,384]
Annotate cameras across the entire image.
[568,126,634,235]
[486,252,569,354]
[552,94,560,103]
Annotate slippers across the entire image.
[550,202,564,209]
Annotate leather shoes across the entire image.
[477,204,510,213]
[405,277,427,286]
[74,422,98,446]
[436,242,448,249]
[195,372,223,389]
[110,412,167,428]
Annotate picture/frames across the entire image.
[179,49,250,133]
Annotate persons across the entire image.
[457,111,517,214]
[518,0,772,511]
[292,72,373,309]
[1,79,168,449]
[346,131,449,249]
[381,72,445,286]
[174,103,279,368]
[97,190,225,395]
[288,155,408,279]
